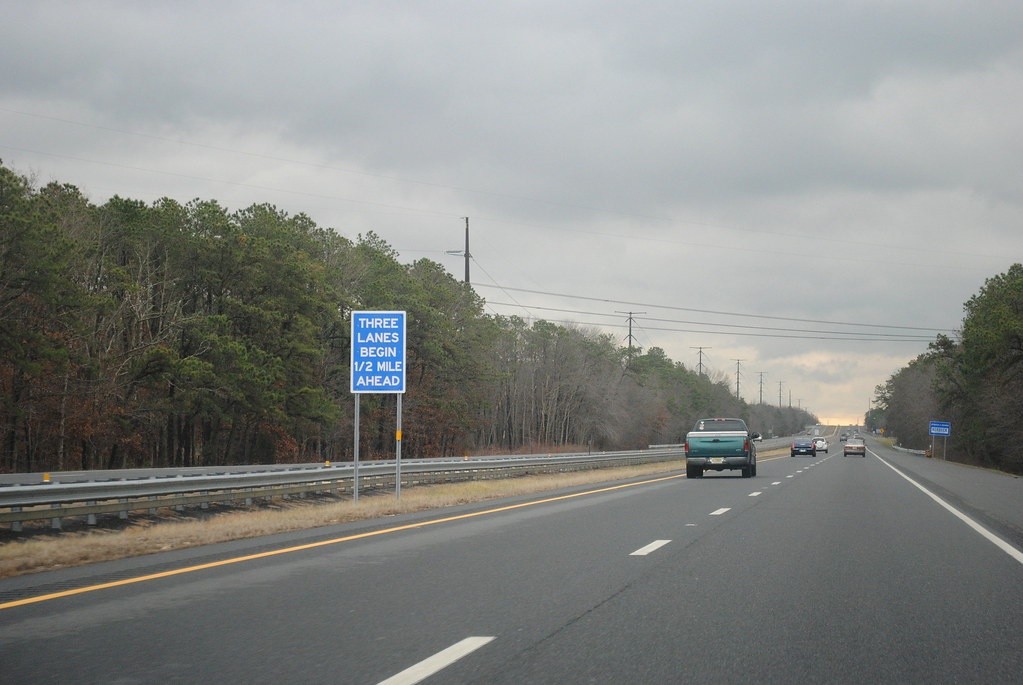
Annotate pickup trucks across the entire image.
[685,417,760,477]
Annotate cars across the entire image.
[813,436,829,453]
[840,434,847,441]
[843,438,867,458]
[791,437,817,458]
[854,434,859,438]
